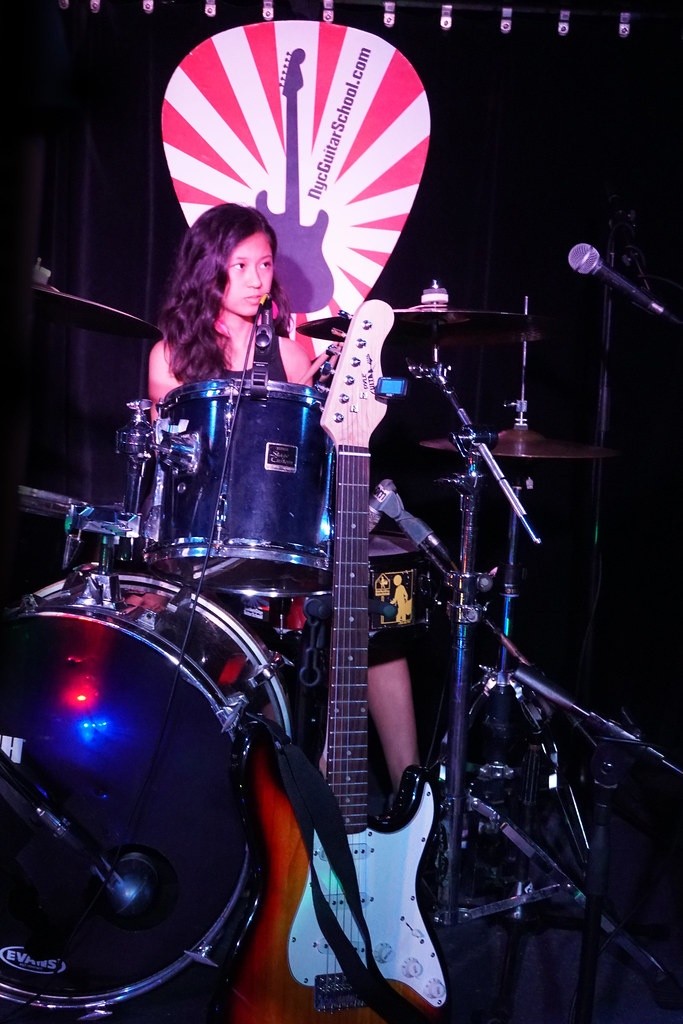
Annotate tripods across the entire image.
[408,314,683,1012]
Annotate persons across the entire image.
[146,203,422,809]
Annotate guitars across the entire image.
[230,299,447,1024]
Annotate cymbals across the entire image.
[295,304,572,348]
[418,422,624,459]
[30,286,163,343]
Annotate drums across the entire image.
[160,380,335,598]
[270,530,438,642]
[12,482,95,571]
[0,562,292,1015]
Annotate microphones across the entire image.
[91,852,164,917]
[367,478,396,534]
[567,242,674,320]
[255,295,278,361]
